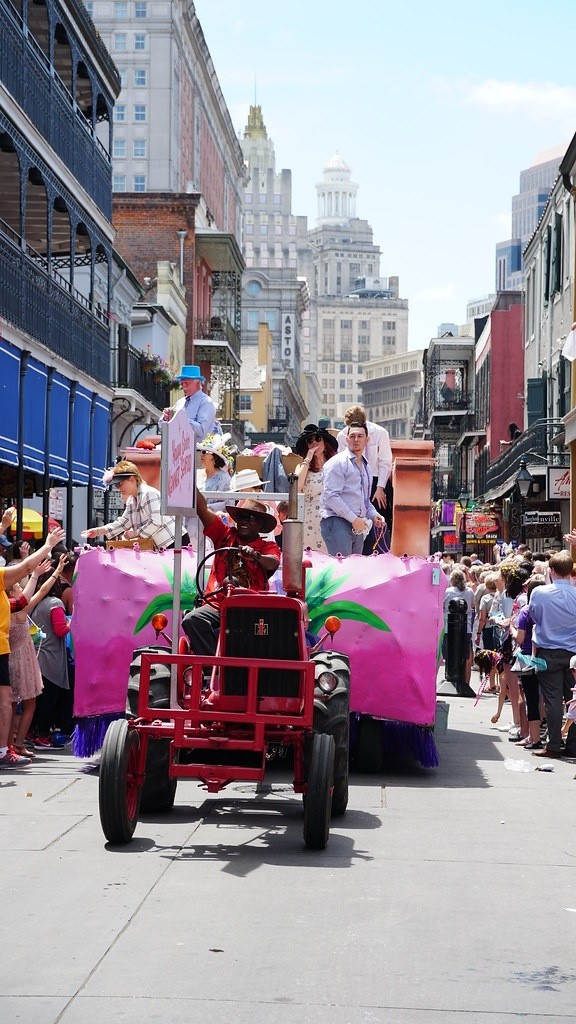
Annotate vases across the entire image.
[153,375,162,382]
[141,364,152,372]
[165,386,174,392]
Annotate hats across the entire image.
[108,471,137,485]
[0,535,12,546]
[225,498,276,533]
[196,443,228,468]
[231,469,269,491]
[176,366,206,381]
[296,423,339,455]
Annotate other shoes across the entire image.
[532,747,562,759]
[483,687,497,694]
[525,741,542,749]
[508,734,523,741]
[497,722,521,735]
[515,738,530,746]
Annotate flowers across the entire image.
[138,344,181,393]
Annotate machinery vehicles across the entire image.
[73,426,439,851]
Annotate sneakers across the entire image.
[0,730,71,769]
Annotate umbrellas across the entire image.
[4,504,62,541]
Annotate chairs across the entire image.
[210,315,224,341]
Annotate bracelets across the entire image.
[301,457,311,466]
[52,576,59,580]
[31,576,38,580]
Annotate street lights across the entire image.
[515,458,536,544]
[458,488,471,556]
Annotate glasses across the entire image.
[202,450,214,455]
[237,513,256,522]
[307,435,322,443]
[115,480,124,489]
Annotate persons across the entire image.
[0,505,106,767]
[182,446,287,549]
[319,420,386,559]
[81,461,191,549]
[159,364,216,444]
[335,405,395,551]
[432,530,576,759]
[295,424,338,557]
[181,487,280,676]
